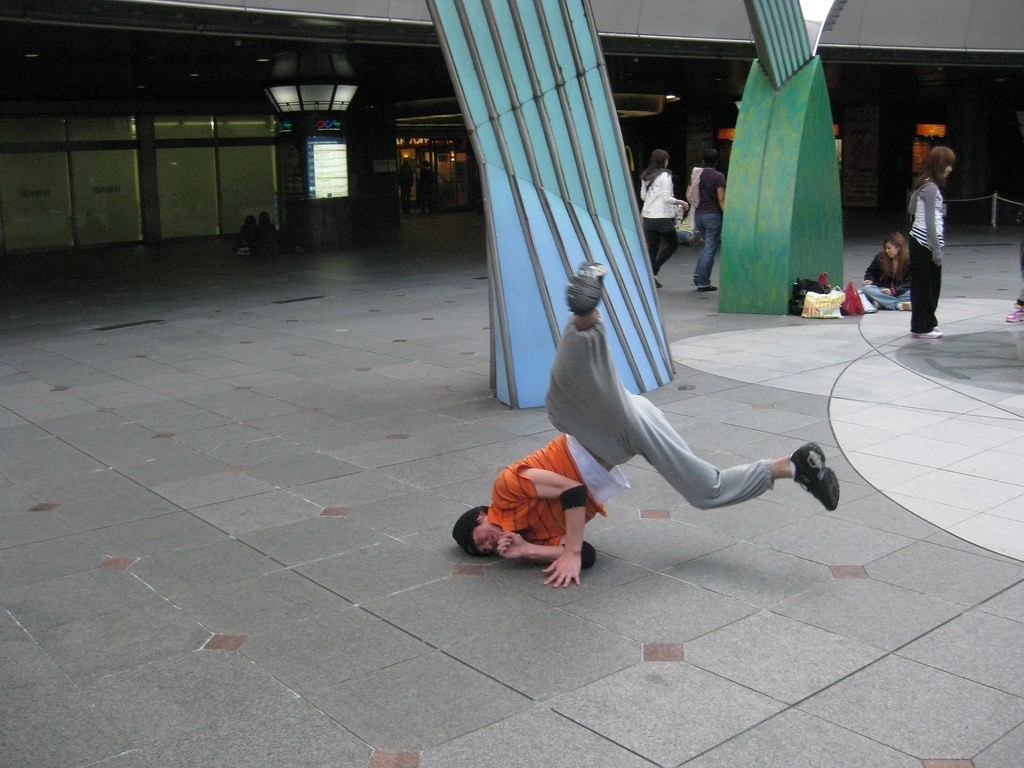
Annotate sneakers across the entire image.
[790,442,839,512]
[1006,310,1024,323]
[566,261,606,313]
[900,301,912,311]
[911,329,942,339]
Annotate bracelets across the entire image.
[890,287,896,297]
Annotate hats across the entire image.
[452,505,490,557]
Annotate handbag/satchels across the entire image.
[801,288,847,319]
[857,289,878,312]
[841,282,863,315]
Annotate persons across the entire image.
[637,149,691,290]
[861,231,912,311]
[1004,240,1024,325]
[907,146,956,341]
[685,146,728,293]
[234,214,260,254]
[416,159,435,215]
[256,210,277,254]
[398,158,413,216]
[446,257,843,589]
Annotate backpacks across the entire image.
[787,278,822,316]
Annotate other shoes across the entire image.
[696,285,717,292]
